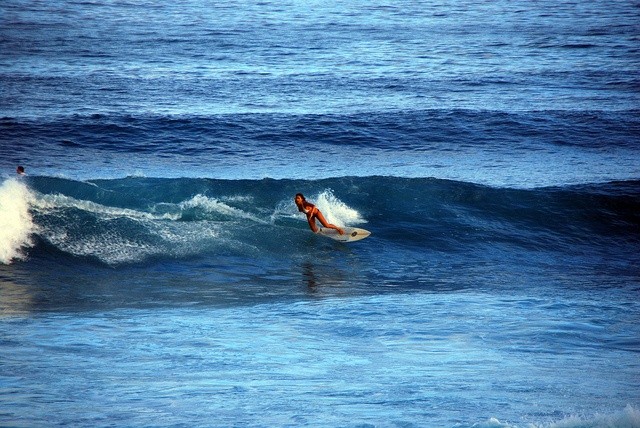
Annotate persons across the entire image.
[294,193,344,236]
[16,165,27,176]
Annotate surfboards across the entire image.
[318,227,371,243]
